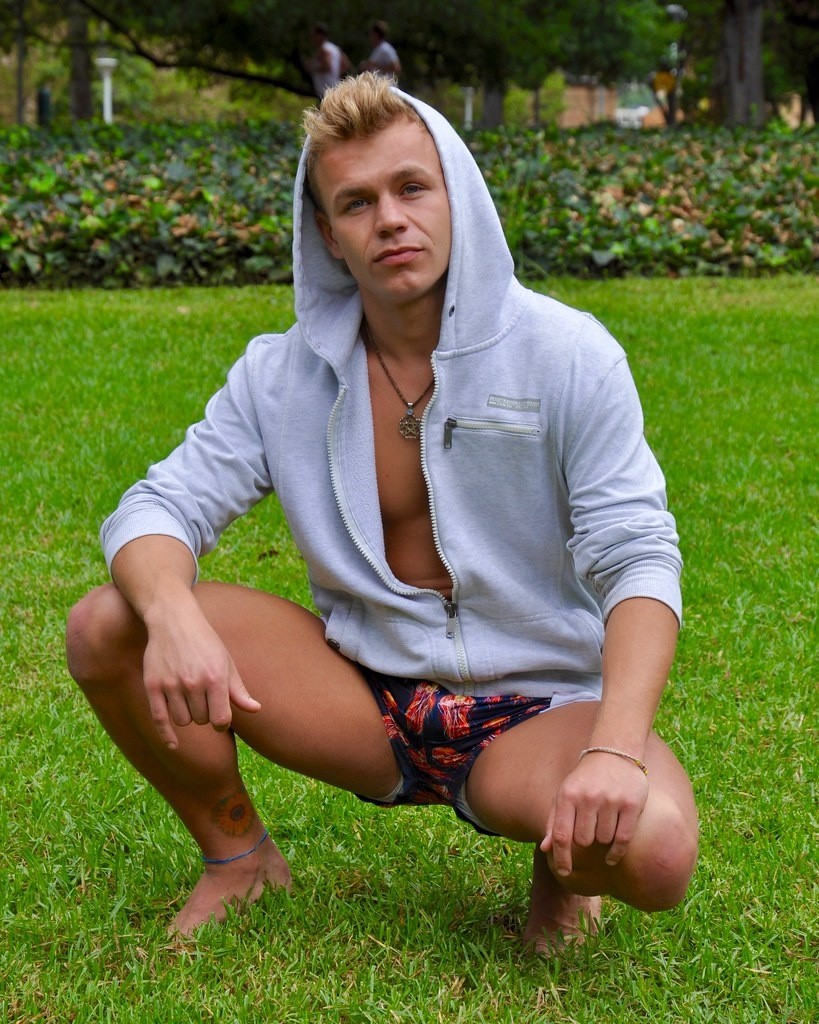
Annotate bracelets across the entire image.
[579,746,649,775]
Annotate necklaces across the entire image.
[367,328,436,440]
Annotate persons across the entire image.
[302,23,343,99]
[68,74,698,960]
[360,17,401,88]
